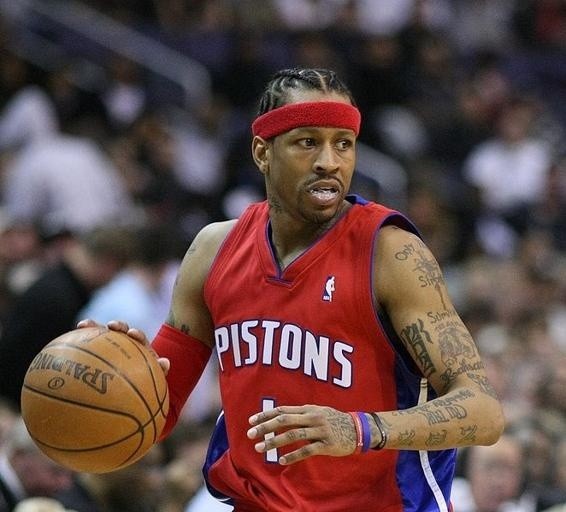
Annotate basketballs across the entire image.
[22,326,171,475]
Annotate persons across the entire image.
[1,0,566,512]
